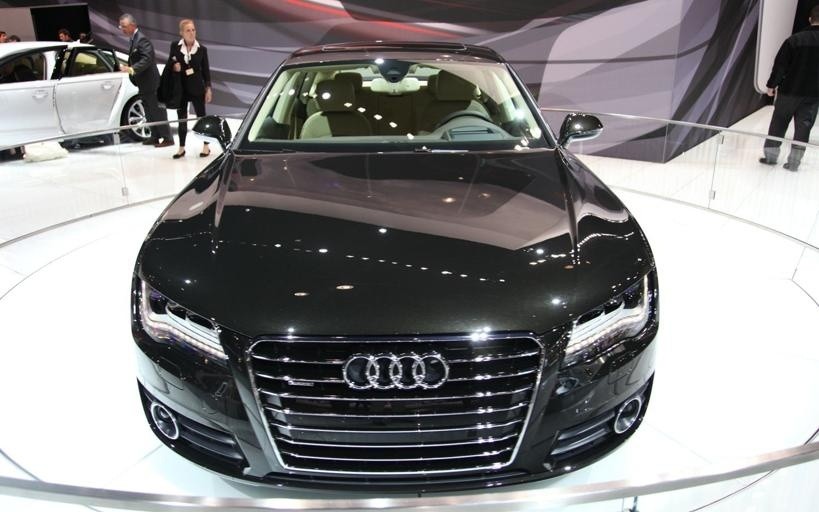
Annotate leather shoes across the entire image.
[143,137,174,147]
[759,157,798,172]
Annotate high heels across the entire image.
[199,149,211,157]
[174,151,185,159]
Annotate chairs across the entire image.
[10,55,110,81]
[298,71,493,140]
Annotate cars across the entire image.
[131,40,661,495]
[0,39,191,152]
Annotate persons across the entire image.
[0,30,20,43]
[58,27,75,42]
[170,19,212,158]
[119,13,174,147]
[760,5,819,172]
